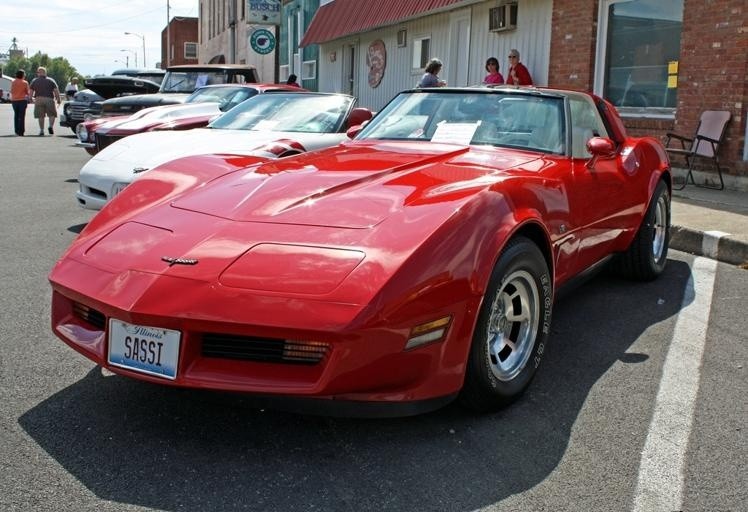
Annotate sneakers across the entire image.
[48,126,54,134]
[40,133,44,136]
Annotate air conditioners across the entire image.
[487,3,516,34]
[396,28,406,48]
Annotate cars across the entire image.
[605,64,677,109]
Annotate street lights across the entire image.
[116,30,148,69]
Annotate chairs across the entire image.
[664,109,735,192]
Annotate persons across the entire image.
[506,49,533,86]
[419,58,446,88]
[64,77,79,101]
[482,57,504,85]
[286,74,299,87]
[29,67,61,135]
[9,69,30,136]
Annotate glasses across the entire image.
[487,63,496,66]
[508,55,517,59]
